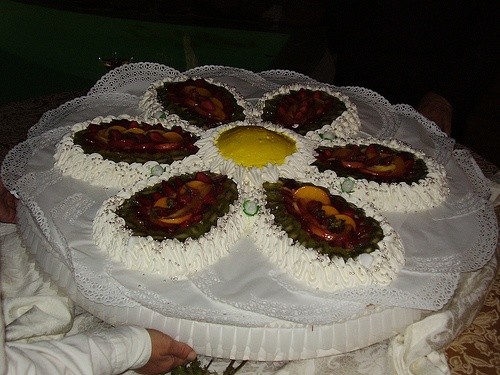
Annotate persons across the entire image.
[284,0,499,138]
[0,183,195,375]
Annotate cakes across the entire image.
[53,73,449,293]
